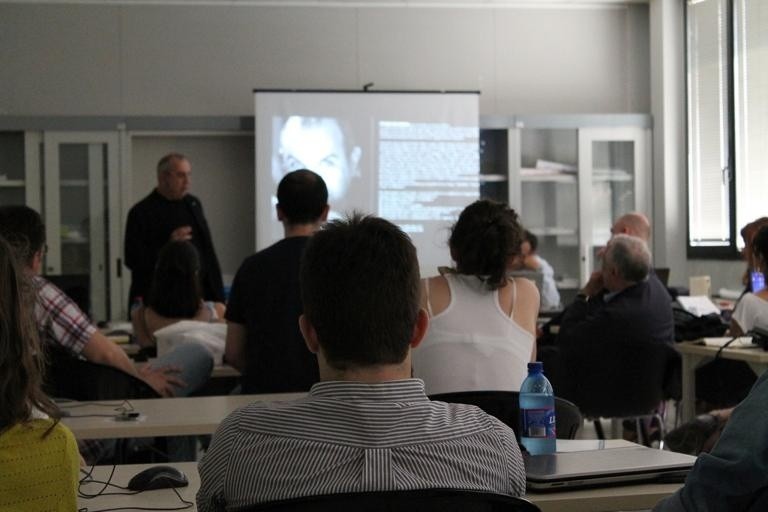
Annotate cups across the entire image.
[688,276,710,296]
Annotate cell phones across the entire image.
[748,267,765,293]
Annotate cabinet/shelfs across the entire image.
[1,127,119,329]
[481,125,652,290]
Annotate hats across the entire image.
[148,343,213,397]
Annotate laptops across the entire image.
[526,447,698,490]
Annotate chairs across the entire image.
[428,389,580,441]
[581,407,666,449]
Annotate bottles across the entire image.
[130,296,143,340]
[517,360,557,476]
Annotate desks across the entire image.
[75,437,700,512]
[669,336,768,424]
[29,393,307,438]
[121,360,243,381]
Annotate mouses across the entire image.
[128,466,189,492]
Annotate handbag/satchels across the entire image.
[664,413,718,456]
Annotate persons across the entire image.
[654,368,768,512]
[124,153,226,363]
[724,220,768,334]
[222,169,330,393]
[2,201,213,470]
[538,213,685,421]
[513,229,559,313]
[197,213,526,512]
[409,200,540,397]
